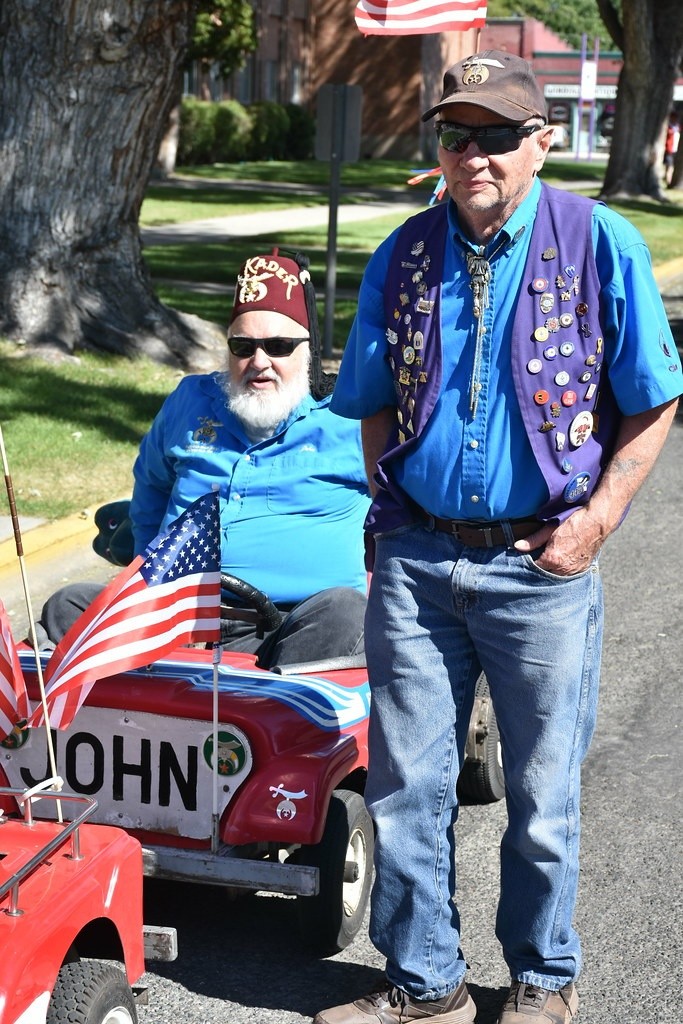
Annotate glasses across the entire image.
[228,337,310,357]
[435,120,541,155]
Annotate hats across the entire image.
[227,247,338,402]
[421,50,548,125]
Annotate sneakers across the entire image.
[313,977,477,1024]
[496,980,579,1024]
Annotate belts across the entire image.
[404,495,545,547]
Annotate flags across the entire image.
[21,490,220,731]
[0,599,33,741]
[353,0,487,37]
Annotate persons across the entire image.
[662,112,681,185]
[39,246,375,674]
[313,52,682,1024]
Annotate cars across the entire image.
[1,571,506,951]
[2,785,180,1024]
